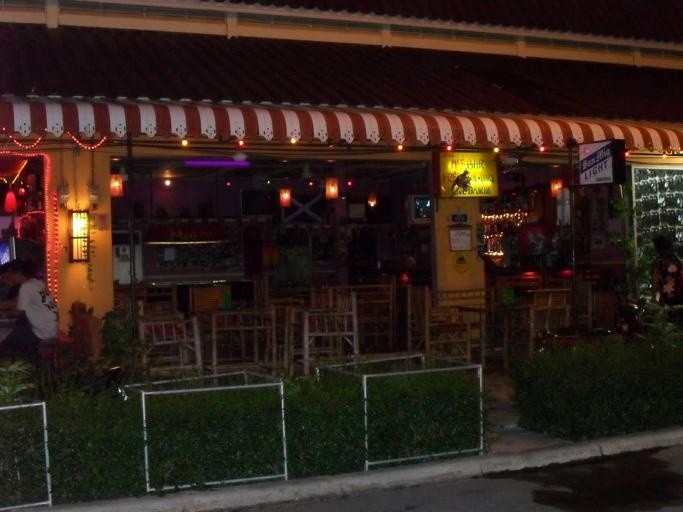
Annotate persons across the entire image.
[649,231,683,325]
[0,258,61,368]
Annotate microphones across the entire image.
[38,338,59,398]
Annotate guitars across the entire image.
[326,174,339,202]
[279,185,292,208]
[367,189,378,210]
[551,177,565,202]
[108,174,123,198]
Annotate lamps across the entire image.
[135,299,203,377]
[521,275,593,345]
[266,286,332,369]
[188,283,245,365]
[288,289,359,377]
[407,283,471,365]
[251,277,304,362]
[142,309,183,321]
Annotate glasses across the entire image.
[4,180,17,212]
[279,176,291,207]
[111,159,122,198]
[324,163,339,198]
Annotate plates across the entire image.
[318,274,398,352]
